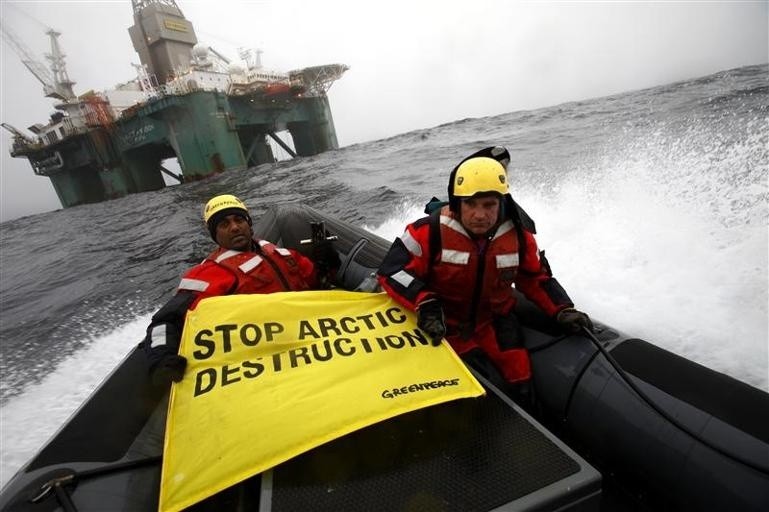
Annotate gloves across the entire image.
[555,307,594,339]
[308,241,343,271]
[156,352,187,383]
[416,305,445,347]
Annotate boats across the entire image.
[0,203,768,512]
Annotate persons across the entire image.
[374,154,594,432]
[140,192,343,385]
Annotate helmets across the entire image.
[454,156,509,197]
[203,194,248,225]
